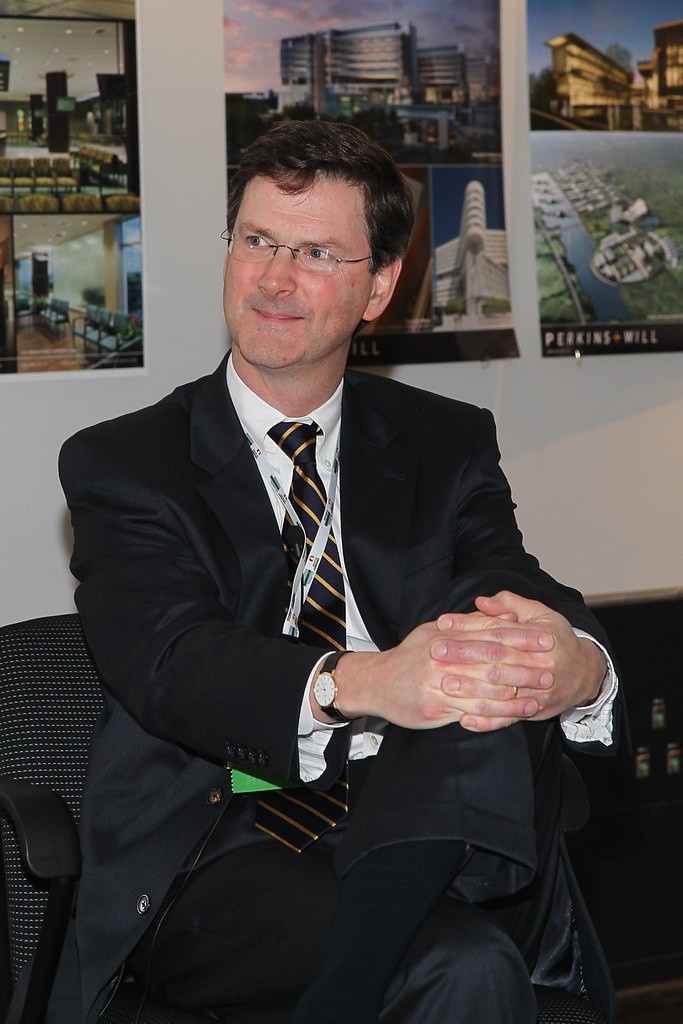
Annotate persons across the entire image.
[3,122,634,1024]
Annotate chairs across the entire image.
[0,611,615,1024]
[0,146,139,211]
[16,296,134,367]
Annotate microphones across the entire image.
[283,525,304,558]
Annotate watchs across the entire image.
[314,651,363,722]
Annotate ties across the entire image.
[254,419,349,855]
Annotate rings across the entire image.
[510,687,518,699]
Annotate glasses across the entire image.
[221,229,374,277]
